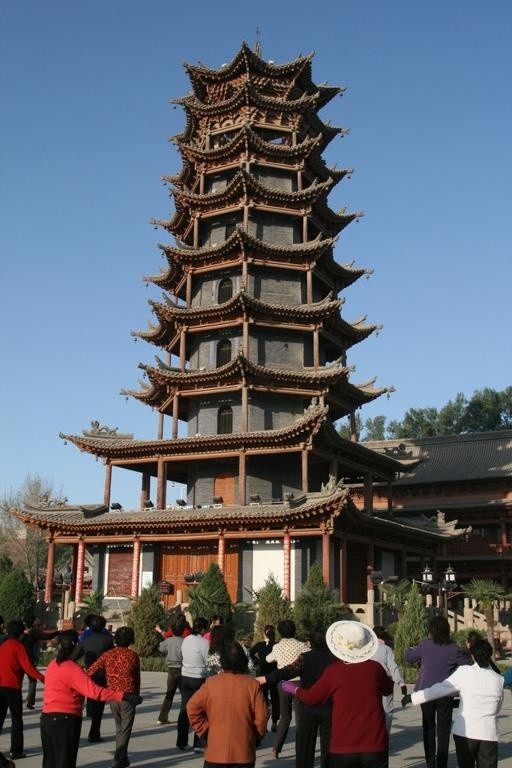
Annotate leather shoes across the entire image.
[9,752,26,760]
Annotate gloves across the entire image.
[400,694,411,708]
[123,692,143,705]
[282,680,298,694]
[400,685,407,695]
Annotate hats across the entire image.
[325,618,379,664]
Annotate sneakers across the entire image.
[271,725,277,732]
[25,703,35,710]
[176,744,192,752]
[194,747,205,753]
[156,720,169,725]
[271,748,279,759]
[88,737,103,744]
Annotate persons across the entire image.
[19,618,61,709]
[40,628,143,768]
[205,625,254,678]
[405,616,468,768]
[0,616,42,759]
[186,640,268,768]
[155,614,190,638]
[266,620,312,760]
[176,617,210,753]
[281,618,393,767]
[251,625,278,730]
[464,631,480,650]
[401,639,505,768]
[157,625,184,725]
[85,625,141,768]
[79,614,114,642]
[368,625,408,734]
[203,614,223,640]
[71,615,114,743]
[254,627,336,768]
